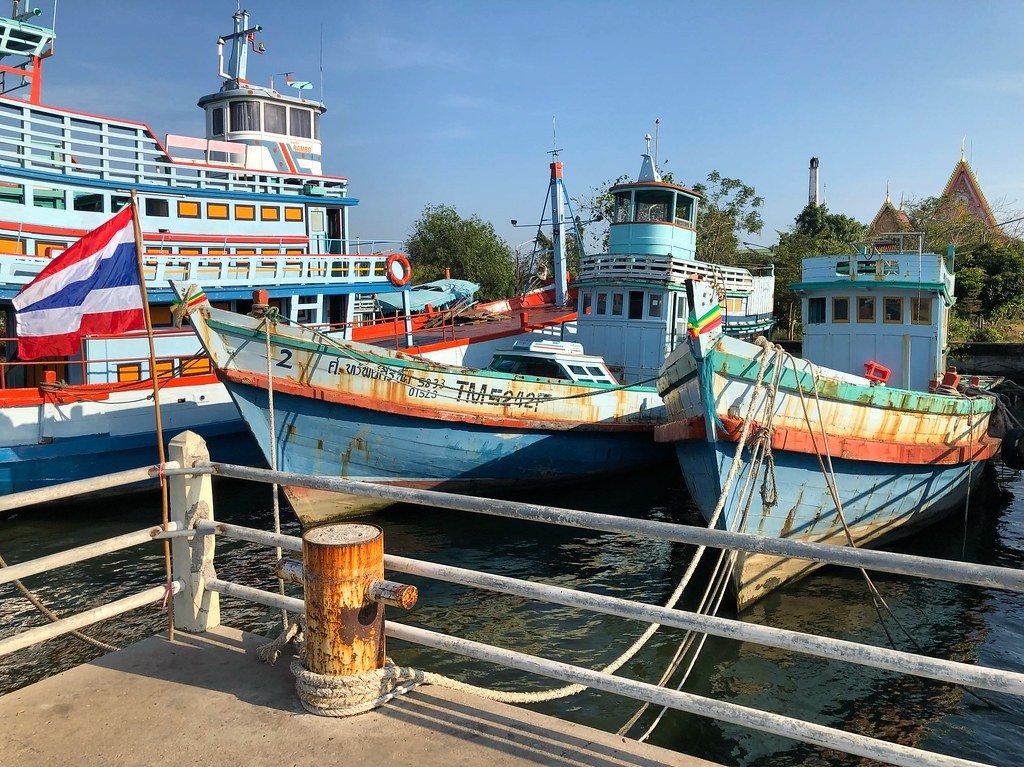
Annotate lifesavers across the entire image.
[1000,429,1024,471]
[387,254,413,286]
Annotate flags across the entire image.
[12,195,153,361]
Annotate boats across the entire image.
[167,111,780,532]
[654,232,1005,620]
[0,4,602,533]
